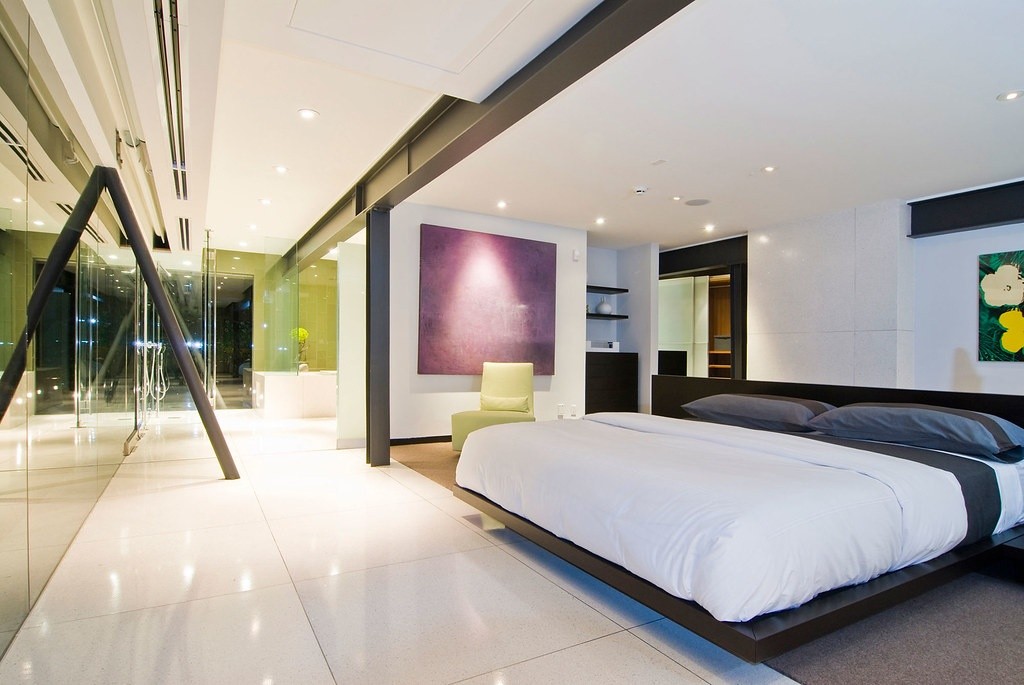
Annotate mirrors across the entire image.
[658,272,732,378]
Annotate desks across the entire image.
[242,367,252,397]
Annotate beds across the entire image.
[454,390,1024,666]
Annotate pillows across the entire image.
[481,395,529,412]
[681,394,837,433]
[805,402,1024,464]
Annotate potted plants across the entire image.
[289,328,309,373]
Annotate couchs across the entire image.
[451,362,536,452]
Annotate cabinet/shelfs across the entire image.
[586,285,629,320]
[658,349,688,376]
[585,352,639,415]
